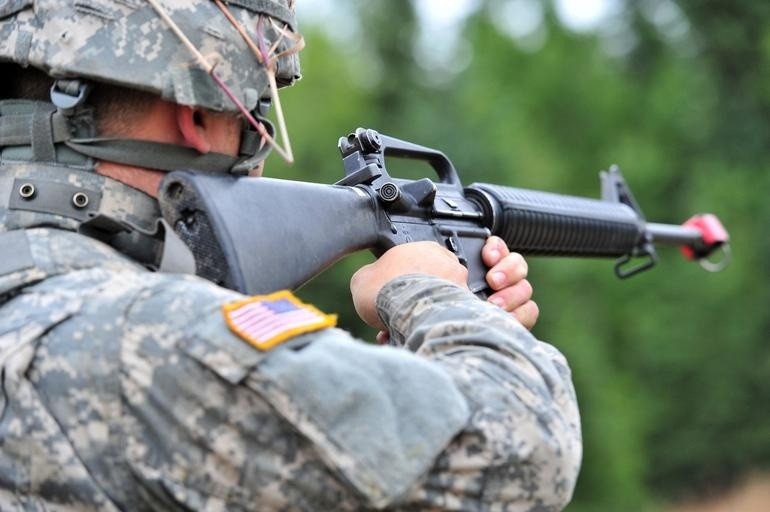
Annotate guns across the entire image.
[158,126,729,304]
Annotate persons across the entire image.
[0,0,583,512]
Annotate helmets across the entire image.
[0,0,301,114]
